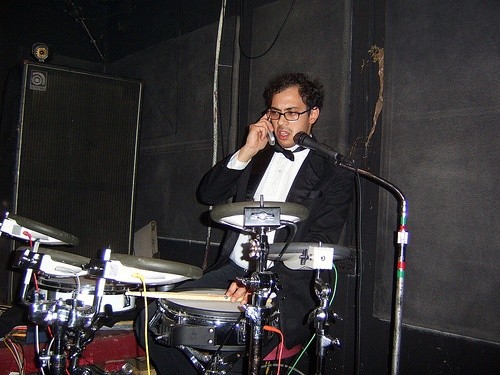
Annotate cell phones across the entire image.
[265,116,275,146]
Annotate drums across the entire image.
[34,274,139,317]
[146,285,253,353]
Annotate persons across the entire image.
[138,72,357,375]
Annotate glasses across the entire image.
[265,107,315,121]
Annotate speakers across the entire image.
[0,60,145,259]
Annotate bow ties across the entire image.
[270,141,307,161]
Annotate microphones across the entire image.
[293,130,353,166]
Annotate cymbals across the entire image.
[12,246,92,277]
[103,252,204,288]
[208,199,310,236]
[266,241,353,271]
[0,212,81,250]
